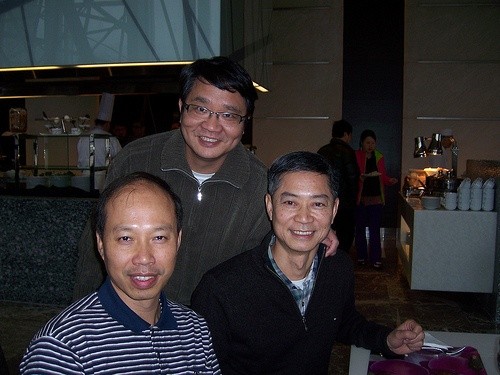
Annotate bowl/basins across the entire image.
[421,179,495,212]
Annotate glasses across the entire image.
[182,100,250,127]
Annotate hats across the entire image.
[95,93,114,122]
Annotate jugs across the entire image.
[418,176,444,191]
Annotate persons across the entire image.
[73,56,339,301]
[77,92,122,194]
[19,171,221,375]
[357,130,398,267]
[317,120,362,249]
[190,151,425,375]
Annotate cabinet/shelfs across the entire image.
[398,192,497,293]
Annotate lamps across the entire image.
[413,132,458,169]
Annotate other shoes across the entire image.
[373,262,385,272]
[356,258,368,265]
